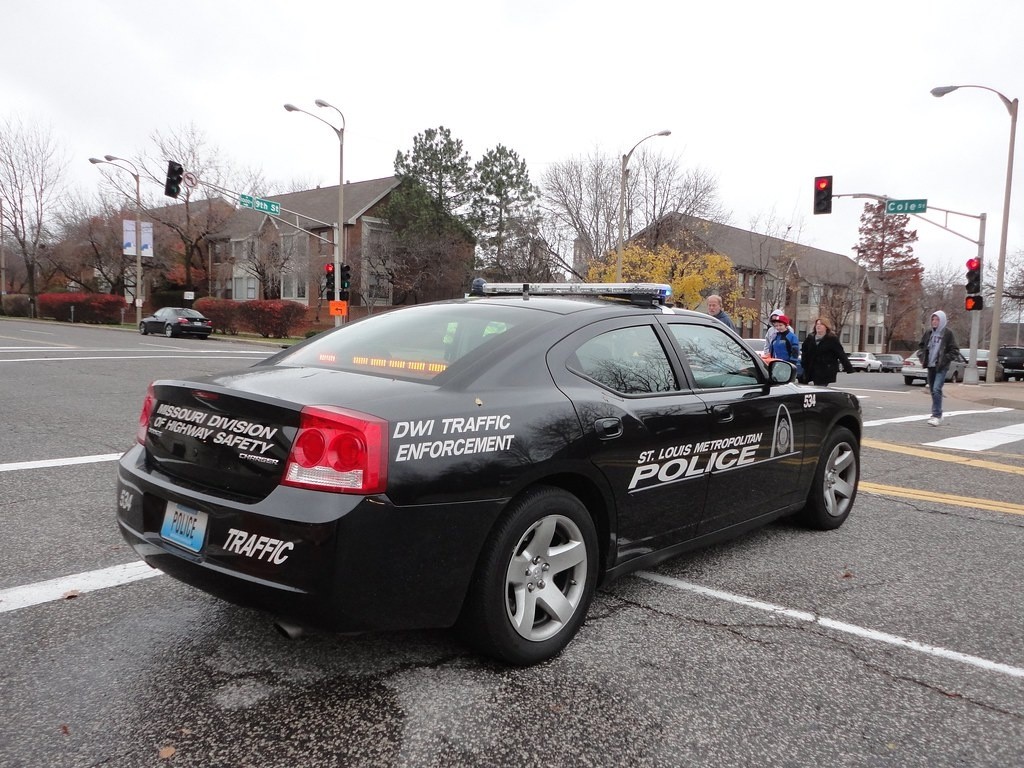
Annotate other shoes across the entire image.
[927,417,944,426]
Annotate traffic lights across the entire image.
[326,262,334,289]
[341,263,351,288]
[327,290,335,301]
[965,296,983,311]
[965,257,980,293]
[164,161,183,200]
[339,290,349,300]
[814,176,831,214]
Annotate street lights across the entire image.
[617,129,671,282]
[284,99,346,326]
[89,155,141,326]
[930,84,1019,383]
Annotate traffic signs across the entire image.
[254,197,280,215]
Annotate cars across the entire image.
[876,355,904,373]
[742,338,801,376]
[902,349,968,385]
[847,352,884,371]
[116,281,863,668]
[138,306,212,340]
[959,348,1005,382]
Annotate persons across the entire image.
[769,315,799,365]
[802,318,854,387]
[917,310,959,426]
[707,295,736,332]
[764,309,794,356]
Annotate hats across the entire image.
[770,316,789,325]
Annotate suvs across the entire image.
[997,347,1024,381]
